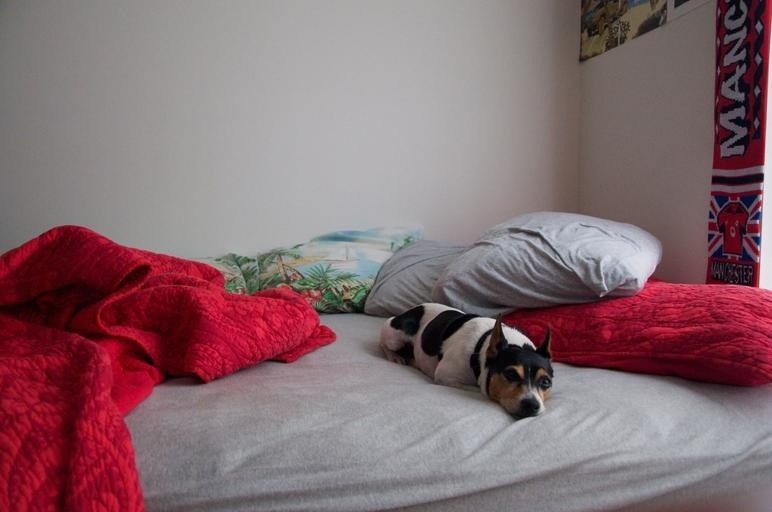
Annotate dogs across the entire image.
[378,302,555,420]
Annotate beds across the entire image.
[0,305,772,511]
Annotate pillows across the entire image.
[365,239,470,316]
[434,208,664,318]
[498,275,771,384]
